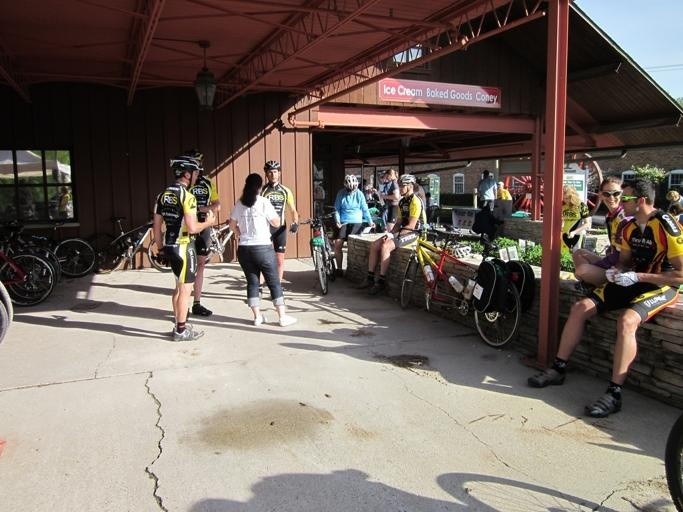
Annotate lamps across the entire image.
[193,40,218,109]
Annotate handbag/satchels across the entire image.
[474,260,504,314]
[506,260,533,313]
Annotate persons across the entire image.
[152,154,216,342]
[259,160,299,295]
[228,172,297,327]
[184,150,225,319]
[476,170,512,210]
[67,192,73,217]
[15,178,34,221]
[58,186,70,220]
[527,176,683,419]
[332,168,430,296]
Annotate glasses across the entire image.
[621,196,637,202]
[602,191,622,197]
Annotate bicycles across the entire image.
[92,214,173,275]
[394,222,535,349]
[0,202,96,344]
[296,210,339,296]
[664,415,683,512]
[202,221,235,265]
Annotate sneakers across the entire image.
[585,393,622,417]
[255,315,267,326]
[173,326,205,341]
[280,316,297,326]
[528,368,566,387]
[369,283,385,294]
[192,304,212,316]
[355,278,373,289]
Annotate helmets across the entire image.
[398,175,416,186]
[264,160,280,172]
[667,191,679,200]
[344,175,358,190]
[168,151,204,178]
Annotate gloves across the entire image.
[606,267,638,287]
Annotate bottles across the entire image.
[448,275,464,294]
[423,262,435,283]
